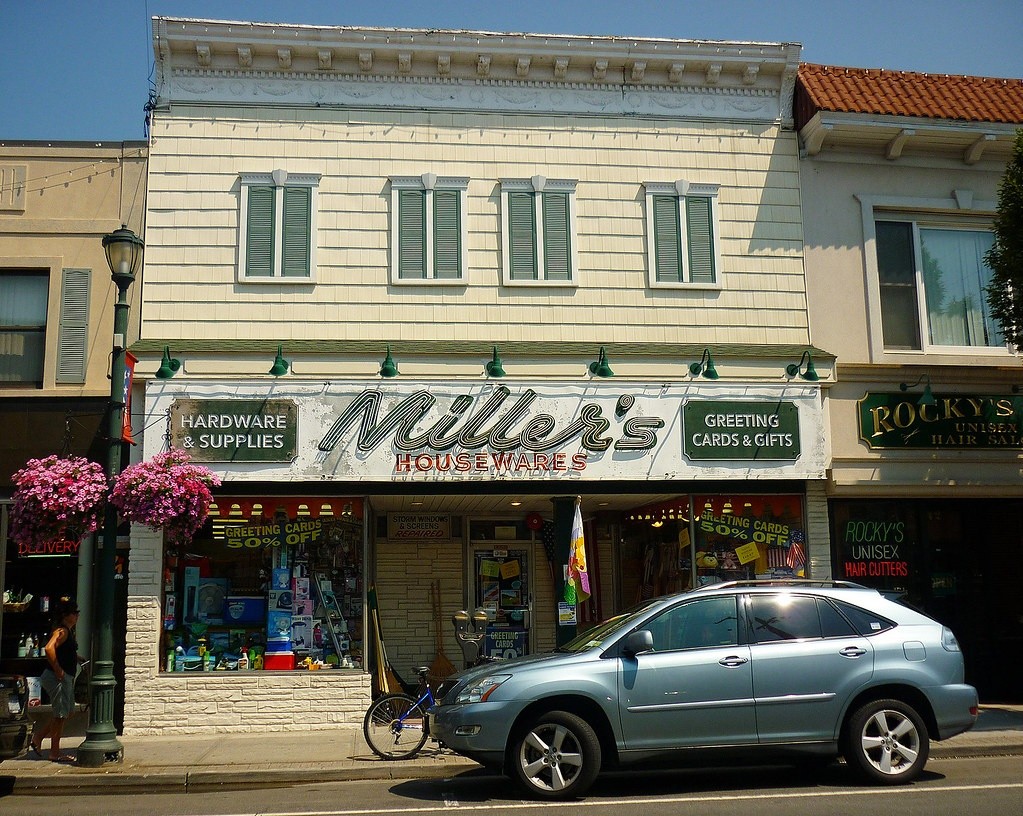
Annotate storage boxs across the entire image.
[263,534,363,673]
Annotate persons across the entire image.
[29,599,91,763]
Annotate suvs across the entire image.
[419,575,980,800]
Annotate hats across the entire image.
[53,591,78,620]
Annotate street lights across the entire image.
[72,220,146,767]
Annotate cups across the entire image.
[341,658,352,666]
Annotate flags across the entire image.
[120,350,140,443]
[566,504,592,603]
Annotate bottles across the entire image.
[18,632,47,657]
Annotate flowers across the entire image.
[8,453,112,556]
[107,444,223,547]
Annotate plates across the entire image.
[339,665,354,669]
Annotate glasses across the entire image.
[71,610,80,614]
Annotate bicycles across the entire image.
[362,655,514,761]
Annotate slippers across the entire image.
[50,755,75,762]
[31,741,42,757]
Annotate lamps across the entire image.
[786,351,821,384]
[151,343,180,380]
[377,345,398,377]
[902,373,939,406]
[589,345,617,379]
[266,342,290,376]
[690,348,720,382]
[483,345,508,380]
